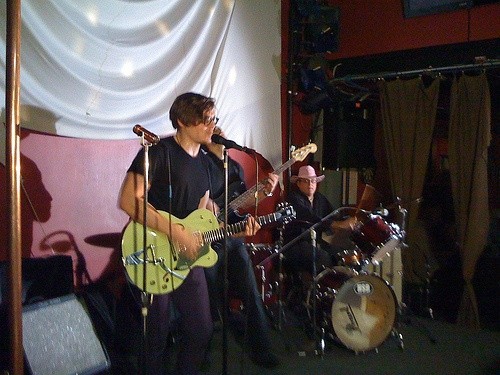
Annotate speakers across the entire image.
[322,102,375,167]
[300,6,340,58]
[0,255,113,375]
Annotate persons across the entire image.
[118,94,280,374]
[282,165,359,280]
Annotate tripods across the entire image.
[263,205,437,359]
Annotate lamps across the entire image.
[288,0,369,123]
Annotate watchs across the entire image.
[261,188,273,196]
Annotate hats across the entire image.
[290,165,325,183]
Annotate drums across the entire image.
[229,241,290,315]
[348,216,400,267]
[305,265,399,354]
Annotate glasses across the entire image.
[299,179,317,183]
[202,117,219,126]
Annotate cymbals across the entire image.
[260,218,313,232]
[384,196,425,211]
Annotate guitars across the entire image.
[207,138,320,252]
[120,201,298,296]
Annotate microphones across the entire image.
[133,125,168,149]
[211,134,255,154]
